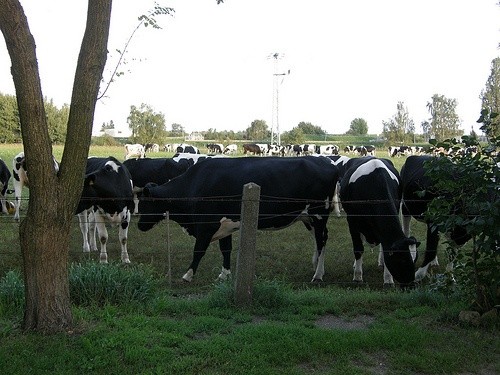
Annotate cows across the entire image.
[399,153,500,279]
[124,139,452,158]
[0,157,16,216]
[13,150,136,263]
[122,155,421,291]
